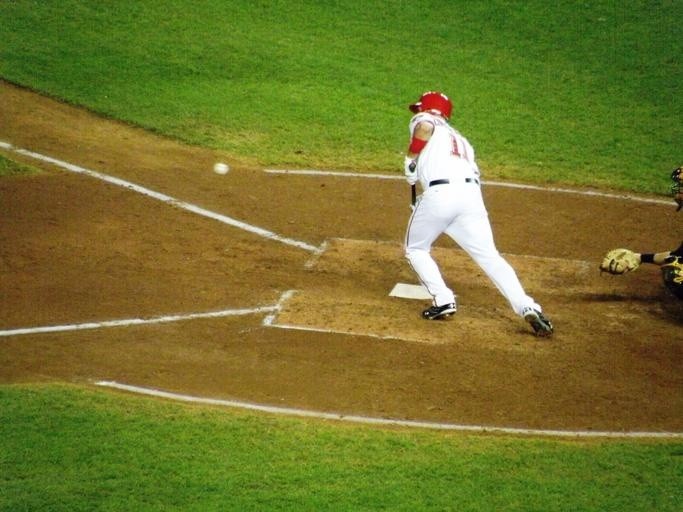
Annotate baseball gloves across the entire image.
[599,248,639,274]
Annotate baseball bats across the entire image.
[409,163,416,205]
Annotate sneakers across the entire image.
[523,307,553,337]
[423,303,457,320]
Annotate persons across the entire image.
[598,166,683,302]
[403,90,554,337]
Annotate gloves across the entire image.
[408,194,423,212]
[404,155,418,186]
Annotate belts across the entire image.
[430,178,479,186]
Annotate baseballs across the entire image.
[213,164,228,174]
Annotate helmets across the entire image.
[409,91,452,121]
[670,165,683,212]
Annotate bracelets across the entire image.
[639,252,655,265]
[409,136,427,153]
